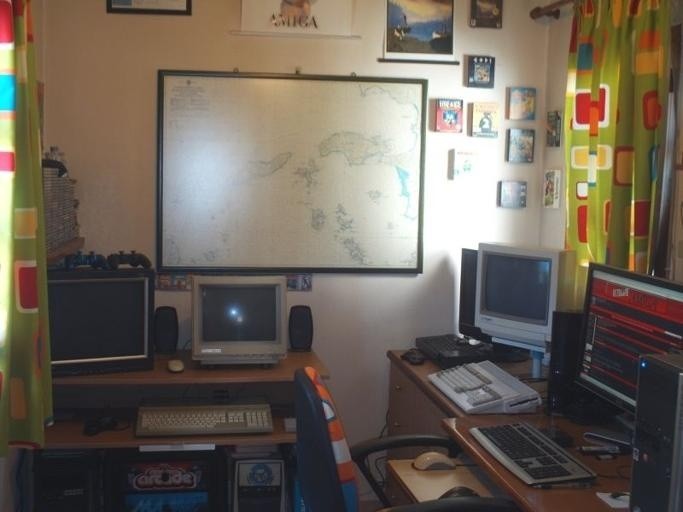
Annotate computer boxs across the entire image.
[228,446,285,512]
[629,353,682,512]
[546,311,597,424]
[34,449,111,511]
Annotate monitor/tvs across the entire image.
[192,276,287,366]
[574,262,682,444]
[458,248,529,362]
[49,269,155,378]
[474,244,577,382]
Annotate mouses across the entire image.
[439,487,479,499]
[413,452,456,471]
[404,348,424,365]
[168,359,184,372]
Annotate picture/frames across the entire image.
[509,127,536,164]
[468,55,495,89]
[377,0,459,63]
[104,1,192,16]
[436,97,463,134]
[230,1,363,42]
[500,179,528,208]
[543,168,562,210]
[453,140,478,179]
[471,101,500,138]
[546,110,562,148]
[509,86,537,121]
[470,0,503,29]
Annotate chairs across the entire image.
[293,365,522,512]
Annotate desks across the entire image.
[384,349,634,512]
[40,348,331,449]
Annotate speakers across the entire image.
[289,305,313,352]
[156,306,178,354]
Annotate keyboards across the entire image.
[470,421,596,486]
[427,360,542,414]
[416,334,488,370]
[136,404,275,436]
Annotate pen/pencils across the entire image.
[575,446,631,454]
